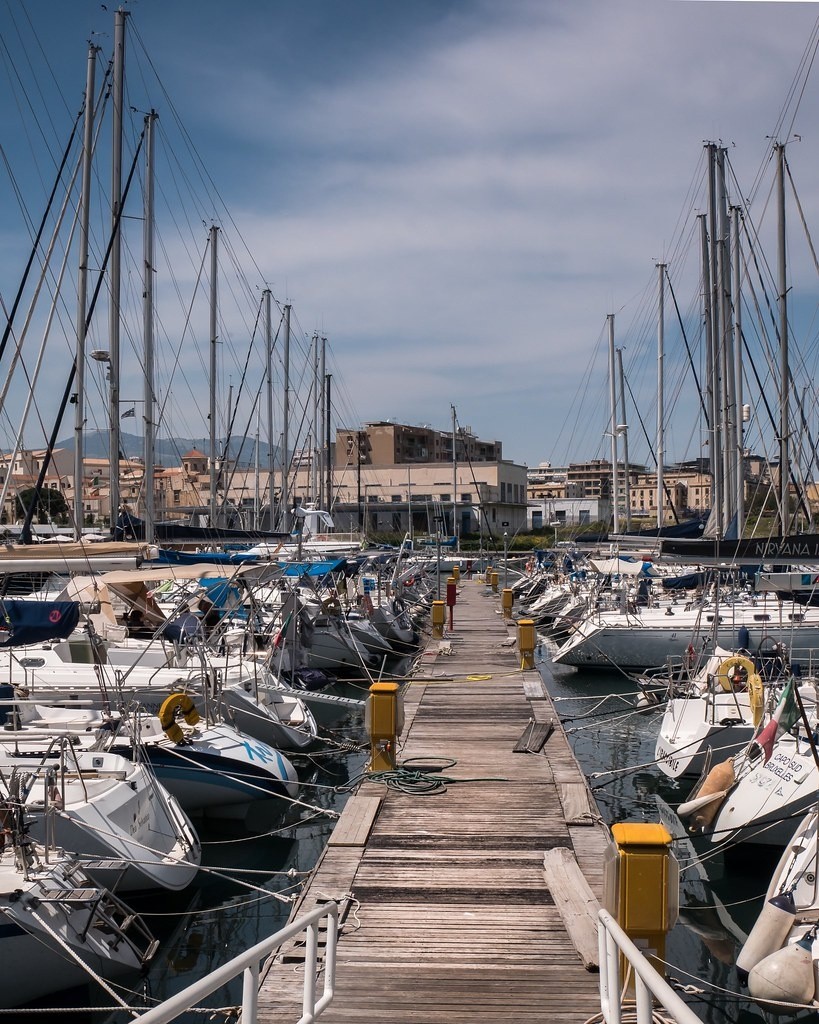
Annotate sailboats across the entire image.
[512,10,819,678]
[0,1,437,895]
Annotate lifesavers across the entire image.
[159,693,200,743]
[322,597,340,615]
[364,594,374,613]
[403,574,415,587]
[718,656,755,692]
[748,674,764,728]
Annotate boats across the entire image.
[419,497,457,545]
[387,556,493,573]
[0,764,159,1010]
[656,650,819,1008]
